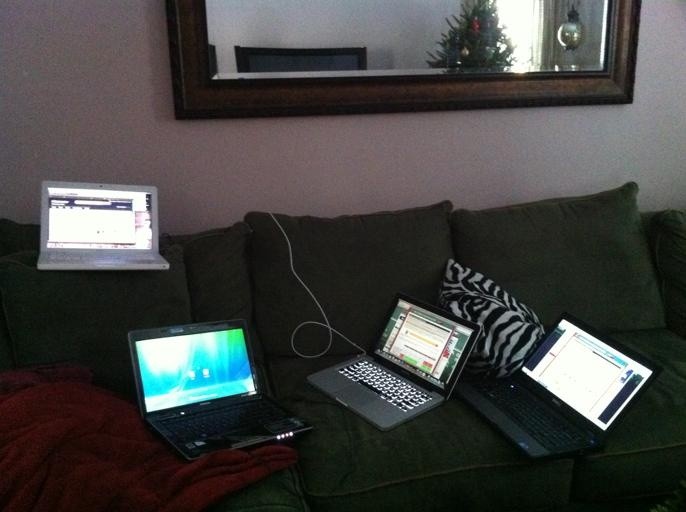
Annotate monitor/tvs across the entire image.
[235,46,367,71]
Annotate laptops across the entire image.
[36,180,171,272]
[454,311,664,460]
[306,291,483,431]
[126,318,313,463]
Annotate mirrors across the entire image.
[165,0,641,119]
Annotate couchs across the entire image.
[132,329,684,512]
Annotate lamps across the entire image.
[557,5,585,51]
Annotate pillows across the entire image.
[3,242,192,374]
[173,219,246,361]
[243,198,455,376]
[437,260,544,386]
[455,180,663,339]
